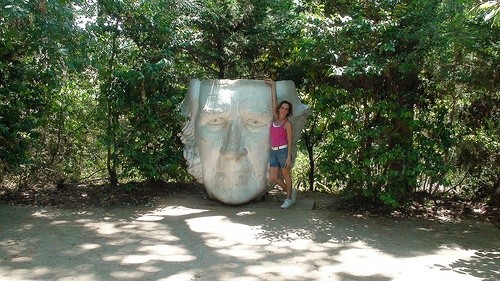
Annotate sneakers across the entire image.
[281,198,294,209]
[291,189,298,203]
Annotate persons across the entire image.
[266,78,295,208]
